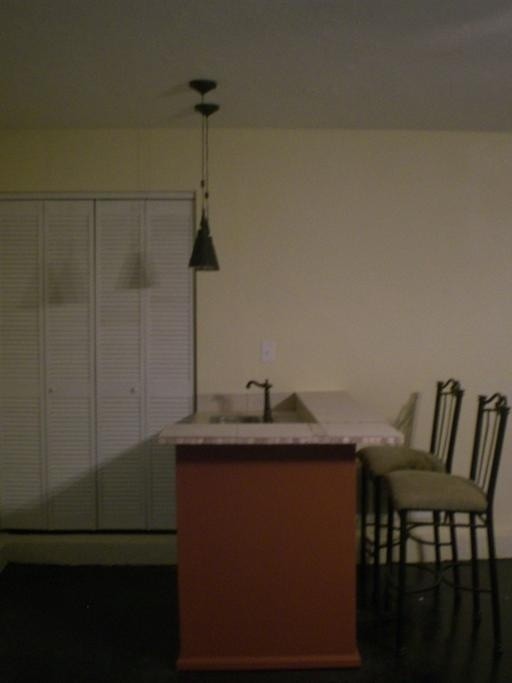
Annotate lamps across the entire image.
[188,80,219,272]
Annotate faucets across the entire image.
[244,377,278,425]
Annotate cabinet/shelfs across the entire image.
[0,198,95,534]
[95,200,196,534]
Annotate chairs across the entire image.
[382,392,510,658]
[356,378,465,612]
